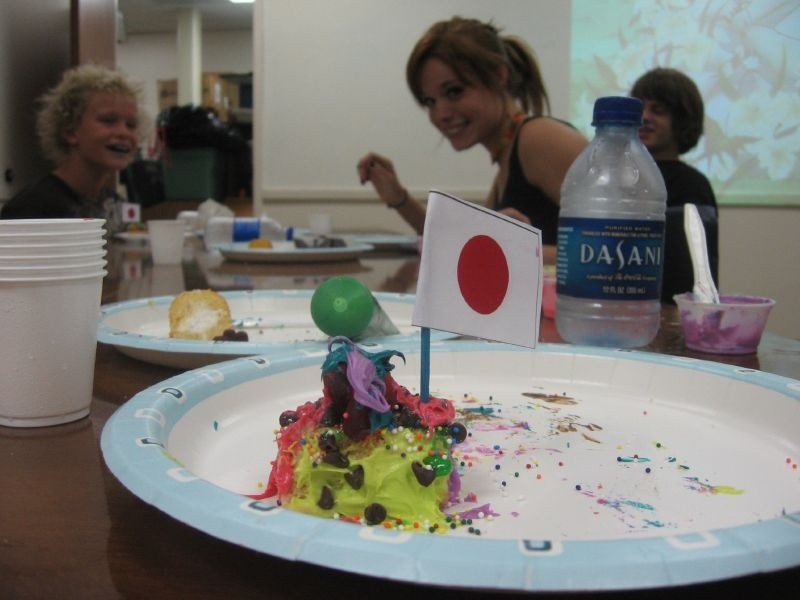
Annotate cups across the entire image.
[311,214,333,233]
[178,210,198,231]
[0,216,108,428]
[147,219,187,265]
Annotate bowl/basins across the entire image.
[673,292,778,355]
[542,263,557,321]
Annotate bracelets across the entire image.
[386,189,408,210]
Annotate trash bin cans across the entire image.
[158,105,226,202]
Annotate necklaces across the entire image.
[489,108,523,164]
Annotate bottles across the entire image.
[204,216,294,252]
[555,95,667,349]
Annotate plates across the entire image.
[212,240,375,262]
[298,233,419,245]
[113,232,193,241]
[97,288,464,371]
[100,340,800,594]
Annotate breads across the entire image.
[168,290,233,340]
[249,239,272,248]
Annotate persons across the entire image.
[630,68,719,306]
[0,67,139,306]
[356,16,590,266]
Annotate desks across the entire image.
[0,252,800,600]
